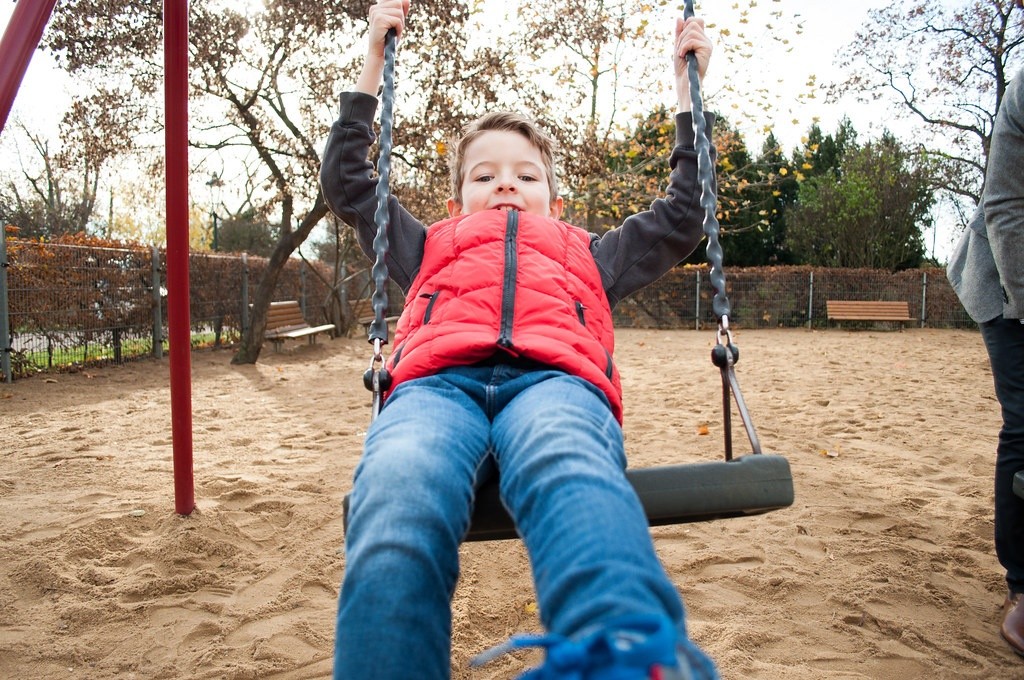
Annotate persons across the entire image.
[945,65,1024,657]
[319,0,713,680]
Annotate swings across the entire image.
[341,0,793,543]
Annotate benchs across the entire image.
[826,301,918,331]
[348,299,400,335]
[248,301,336,353]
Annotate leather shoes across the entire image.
[999,592,1024,655]
